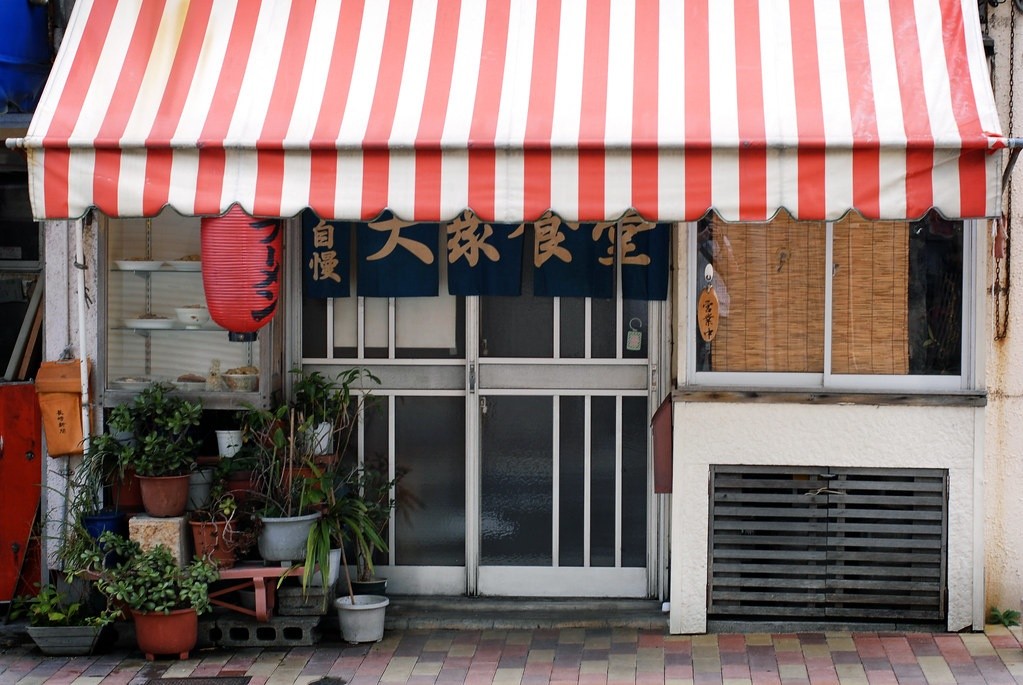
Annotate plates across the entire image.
[119,317,179,330]
[166,260,201,271]
[112,260,165,270]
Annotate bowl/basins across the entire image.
[168,377,207,392]
[221,373,260,393]
[111,377,155,393]
[175,308,210,329]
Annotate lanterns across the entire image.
[200,204,281,342]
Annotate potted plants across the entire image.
[14,368,409,663]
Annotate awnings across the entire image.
[6,1,1023,223]
